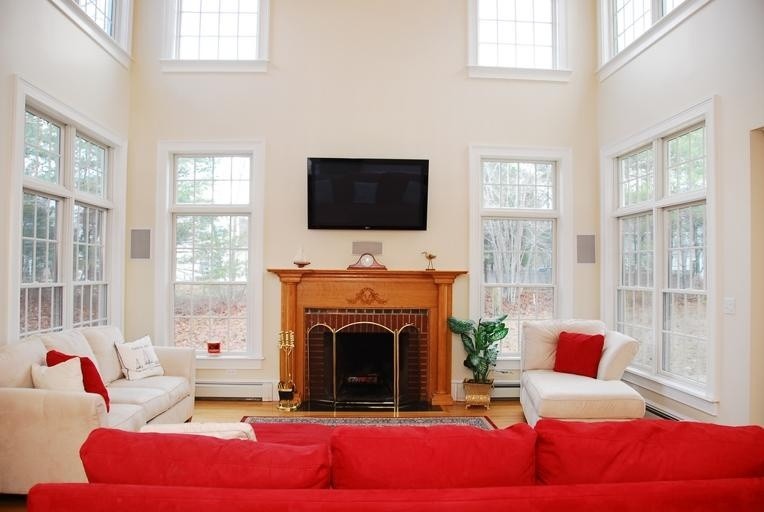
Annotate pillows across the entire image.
[247,421,539,490]
[31,356,86,393]
[45,350,110,413]
[535,417,764,484]
[78,426,332,488]
[553,331,604,378]
[113,335,166,380]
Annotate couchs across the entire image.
[519,318,647,427]
[25,416,764,512]
[0,324,197,495]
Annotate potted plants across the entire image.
[447,314,509,409]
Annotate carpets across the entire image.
[240,415,498,431]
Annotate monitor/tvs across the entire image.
[306,156,430,231]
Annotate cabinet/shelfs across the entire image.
[267,253,468,406]
